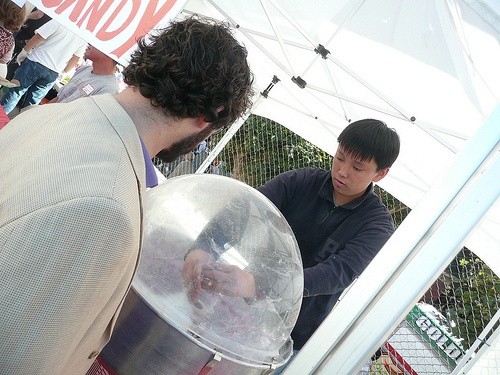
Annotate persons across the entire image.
[1,1,88,116]
[178,118,402,354]
[1,16,254,374]
[45,38,120,104]
